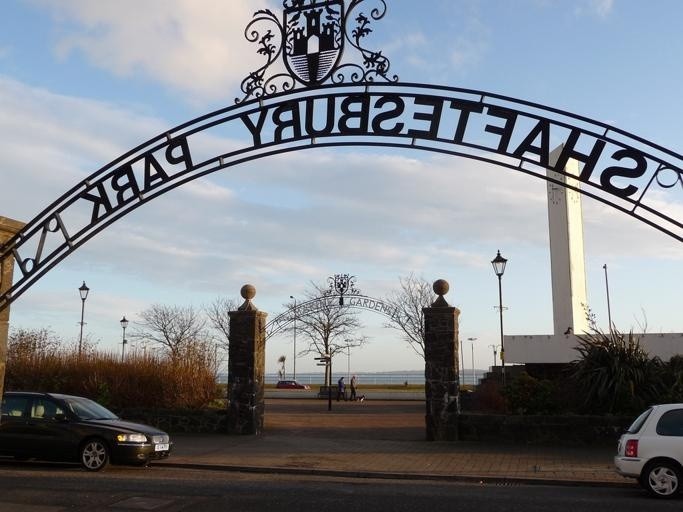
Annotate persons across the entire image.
[349,375,358,401]
[563,326,573,335]
[336,377,347,402]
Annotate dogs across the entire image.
[357,395,365,402]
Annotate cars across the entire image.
[276,380,310,389]
[614,404,682,498]
[0,392,170,472]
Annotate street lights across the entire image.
[121,316,129,361]
[490,252,507,365]
[78,282,88,363]
[489,344,501,365]
[290,296,296,380]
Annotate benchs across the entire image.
[318,386,347,401]
